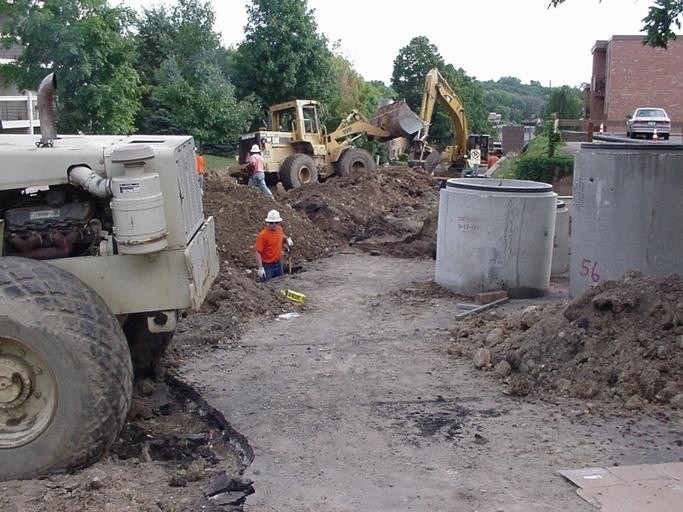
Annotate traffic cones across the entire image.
[651,126,659,142]
[599,122,603,135]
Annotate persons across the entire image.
[247,144,277,202]
[256,210,294,283]
[196,147,206,196]
[460,152,500,178]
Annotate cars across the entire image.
[624,106,672,141]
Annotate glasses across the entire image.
[267,221,279,224]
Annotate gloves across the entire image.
[287,238,293,246]
[258,266,266,281]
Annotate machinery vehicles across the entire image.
[407,66,504,176]
[488,142,503,159]
[0,70,223,485]
[228,96,435,195]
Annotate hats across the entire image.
[250,144,261,153]
[265,210,283,222]
